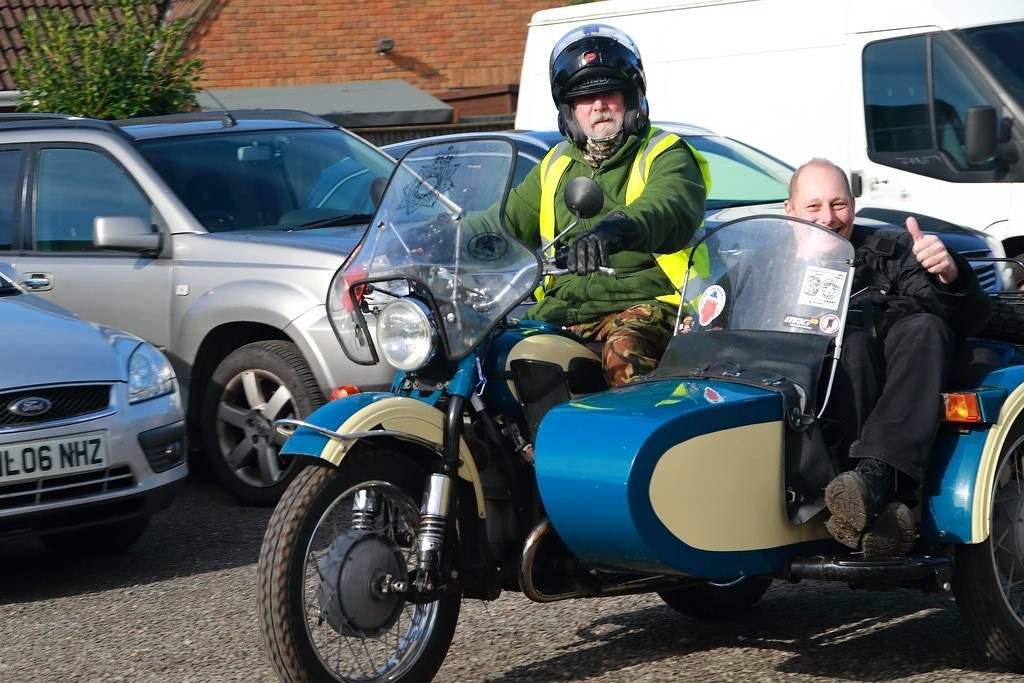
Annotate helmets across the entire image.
[547,26,651,149]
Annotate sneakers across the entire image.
[828,460,896,549]
[858,500,916,561]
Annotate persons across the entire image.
[701,156,993,561]
[387,23,711,396]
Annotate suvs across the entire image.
[1,97,486,500]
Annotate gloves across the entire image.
[386,223,456,275]
[566,214,637,280]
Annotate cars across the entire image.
[1,273,194,552]
[372,120,1010,292]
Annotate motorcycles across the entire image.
[250,133,1024,683]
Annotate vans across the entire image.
[515,0,1021,242]
